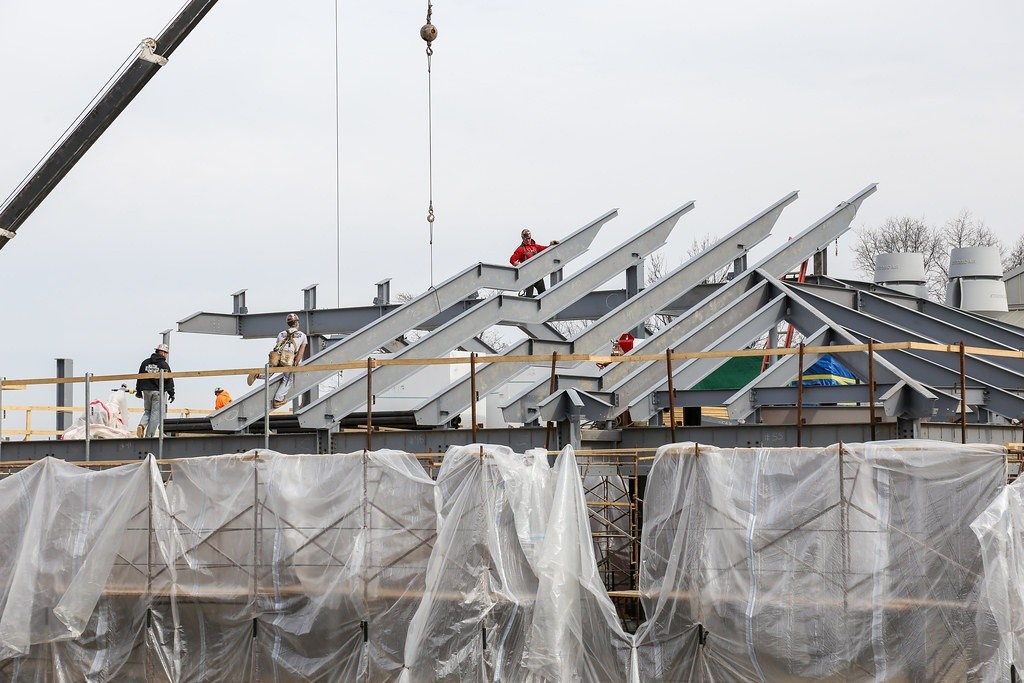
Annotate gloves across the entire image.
[551,240,559,245]
[136,390,142,398]
[168,395,175,403]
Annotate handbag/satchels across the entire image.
[280,350,294,366]
[268,350,280,367]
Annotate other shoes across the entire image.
[137,426,144,437]
[273,398,288,408]
[247,371,259,386]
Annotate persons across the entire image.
[136,344,175,438]
[510,229,559,298]
[247,313,308,408]
[215,387,233,411]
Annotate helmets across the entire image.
[286,313,299,322]
[154,344,169,353]
[521,229,531,239]
[215,387,223,392]
